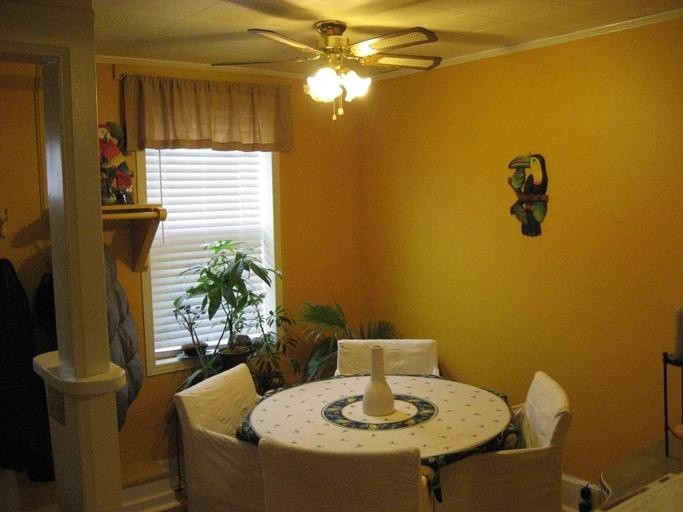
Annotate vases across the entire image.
[361,346,394,417]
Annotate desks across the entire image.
[247,371,516,472]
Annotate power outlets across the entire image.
[579,483,601,512]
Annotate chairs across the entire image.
[331,338,441,379]
[173,360,268,512]
[432,369,573,512]
[258,436,431,512]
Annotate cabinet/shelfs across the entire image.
[660,351,682,458]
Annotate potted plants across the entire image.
[172,238,286,371]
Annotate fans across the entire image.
[205,19,444,75]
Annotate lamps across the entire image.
[300,66,373,121]
